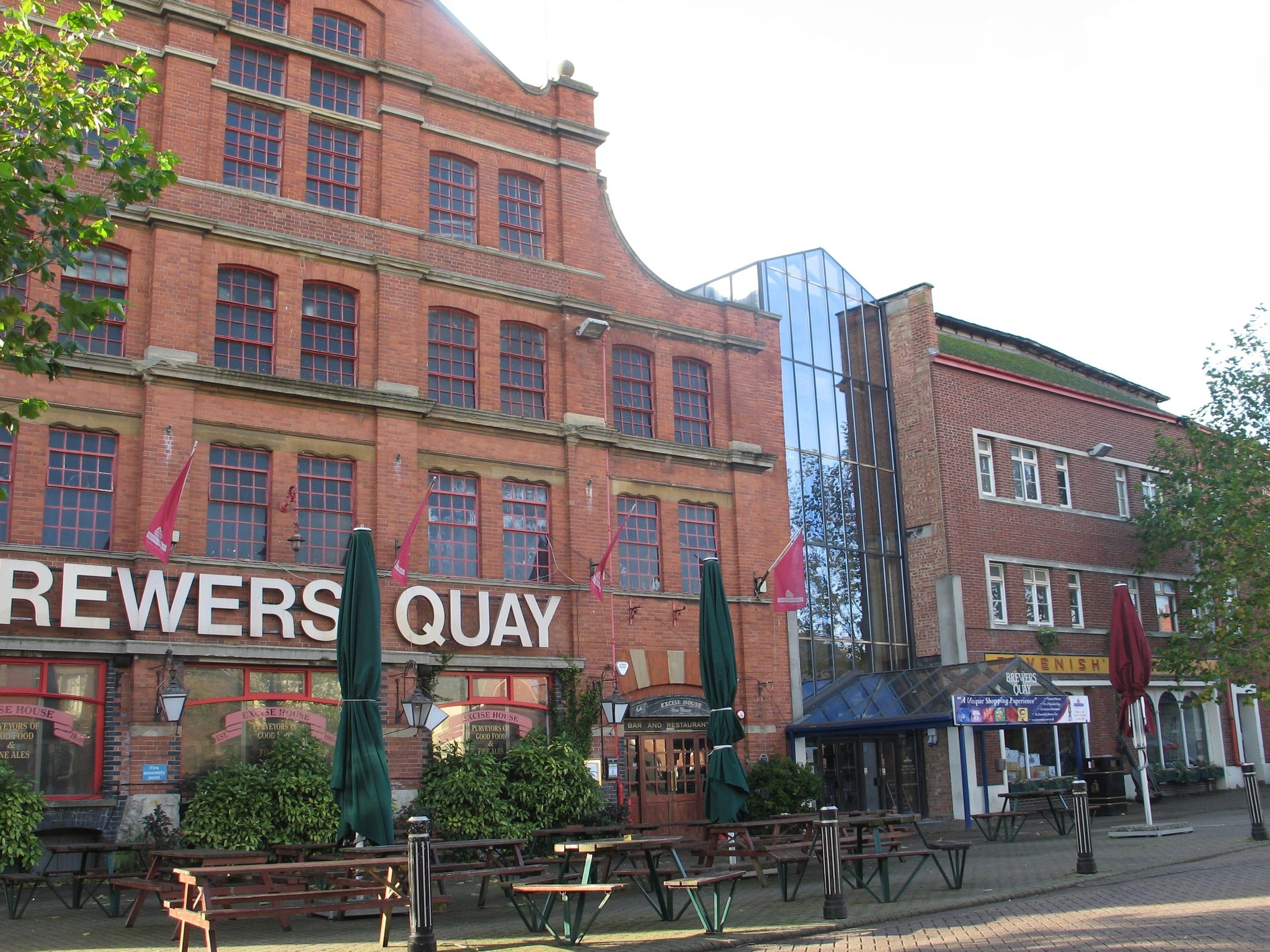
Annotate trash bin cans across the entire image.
[1081,756,1128,816]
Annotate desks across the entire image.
[271,836,446,894]
[125,845,291,932]
[10,840,146,920]
[538,834,715,935]
[992,788,1076,838]
[172,855,409,952]
[335,838,531,921]
[618,819,712,876]
[788,811,953,896]
[701,812,850,888]
[766,808,862,874]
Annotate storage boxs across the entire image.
[1005,747,1056,780]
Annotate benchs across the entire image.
[977,812,1033,817]
[0,827,974,920]
[1065,802,1102,813]
[1028,806,1073,812]
[971,810,1010,818]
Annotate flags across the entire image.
[589,510,632,602]
[391,482,436,587]
[774,530,807,611]
[143,449,196,566]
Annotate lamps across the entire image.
[591,666,632,724]
[394,658,435,731]
[575,314,609,341]
[1088,442,1113,458]
[151,646,191,725]
[278,485,306,551]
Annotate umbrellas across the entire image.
[698,556,747,865]
[1108,583,1153,825]
[336,525,395,900]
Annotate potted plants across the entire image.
[1152,758,1225,784]
[1034,626,1061,652]
